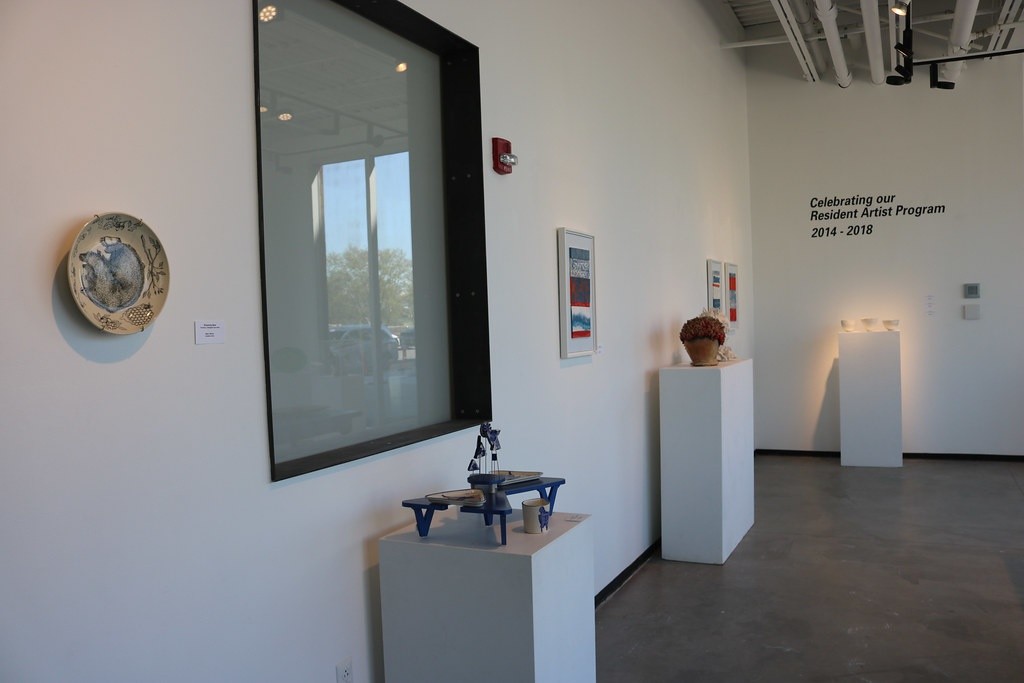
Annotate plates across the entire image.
[490,470,543,486]
[67,212,170,335]
[425,489,487,506]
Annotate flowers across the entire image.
[678,316,726,346]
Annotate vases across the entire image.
[683,338,719,366]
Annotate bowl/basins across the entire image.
[861,319,878,332]
[841,319,858,332]
[883,320,899,331]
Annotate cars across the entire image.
[329,325,401,376]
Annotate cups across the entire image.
[521,498,550,534]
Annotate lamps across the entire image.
[886,0,955,91]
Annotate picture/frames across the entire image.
[708,259,739,329]
[558,227,597,359]
